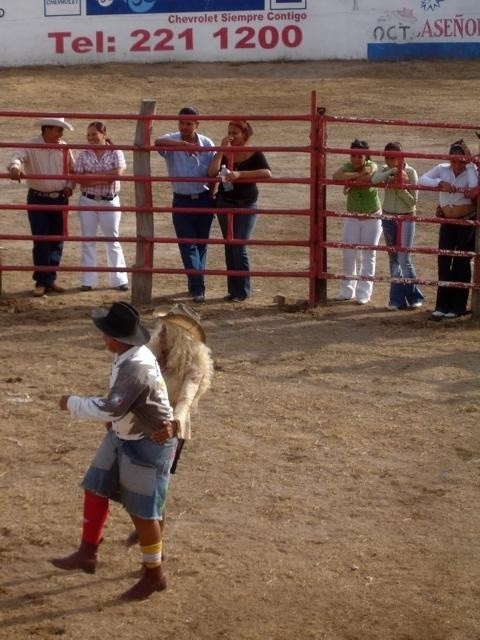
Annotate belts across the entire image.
[31,191,64,199]
[174,193,209,200]
[81,191,117,201]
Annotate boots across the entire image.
[52,541,98,574]
[121,565,168,601]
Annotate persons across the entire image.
[7,117,74,295]
[49,302,179,601]
[126,303,215,569]
[156,109,219,304]
[333,138,384,304]
[208,119,271,302]
[419,132,480,320]
[74,122,128,291]
[370,141,425,311]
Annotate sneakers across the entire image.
[118,284,131,290]
[189,286,250,303]
[32,281,91,296]
[334,293,467,317]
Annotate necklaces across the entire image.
[232,152,245,171]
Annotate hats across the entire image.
[35,112,75,132]
[151,302,207,345]
[90,300,151,346]
